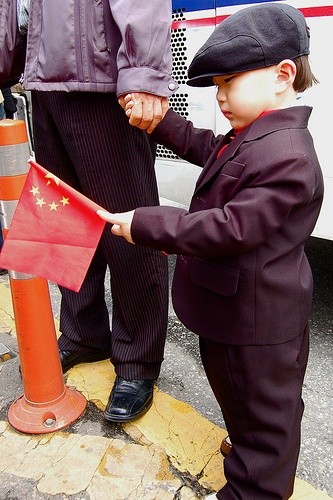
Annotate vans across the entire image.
[0,1,333,244]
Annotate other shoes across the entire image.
[220,437,231,457]
[203,491,218,500]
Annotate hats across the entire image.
[187,2,311,87]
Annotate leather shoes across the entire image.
[103,377,154,421]
[19,340,114,377]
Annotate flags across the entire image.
[0,161,106,292]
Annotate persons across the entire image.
[97,2,325,500]
[0,0,179,423]
[0,88,18,120]
